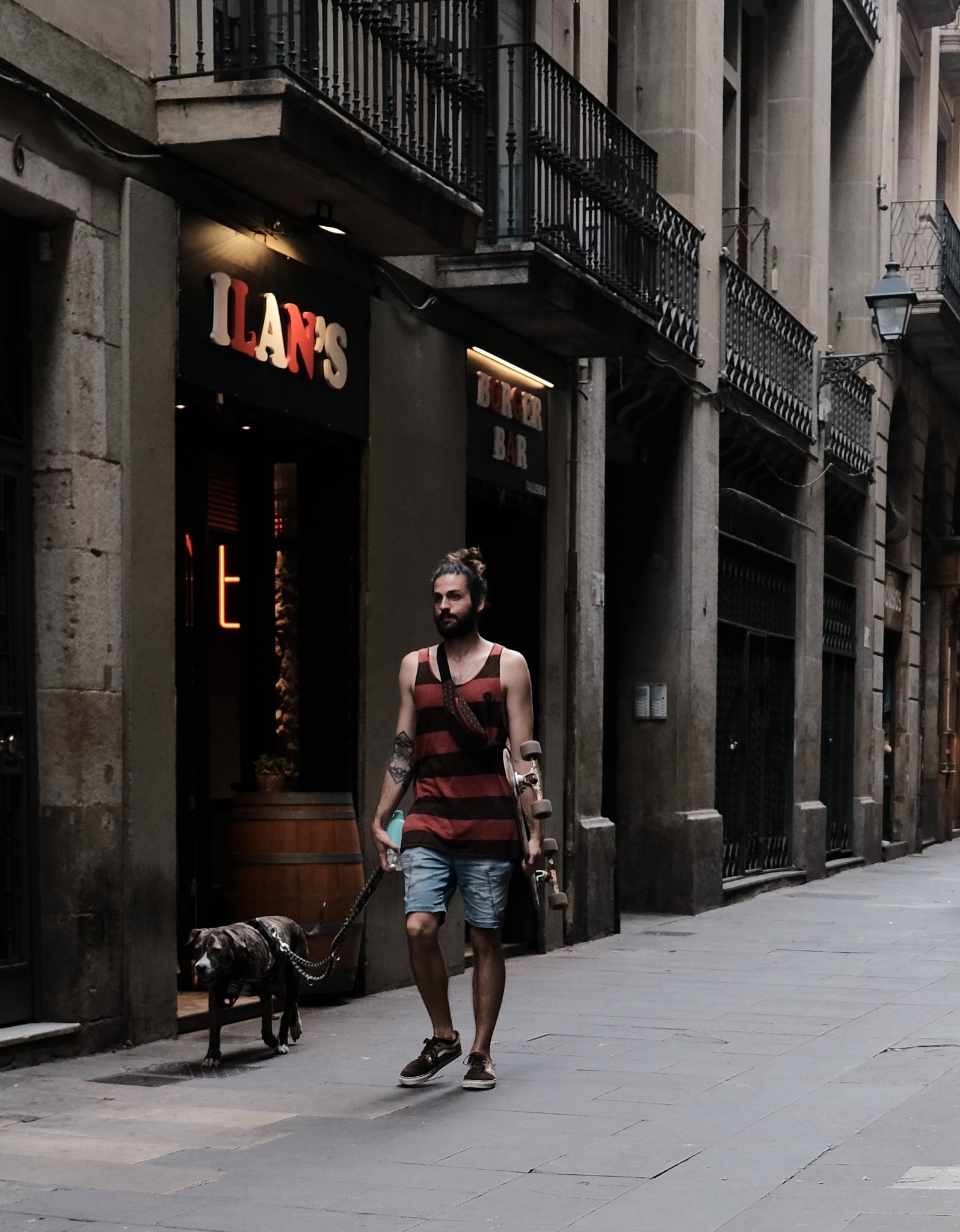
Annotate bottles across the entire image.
[386,810,406,871]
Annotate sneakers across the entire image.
[398,1030,462,1084]
[462,1051,497,1089]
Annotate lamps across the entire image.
[814,256,922,402]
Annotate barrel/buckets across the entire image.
[228,791,365,1001]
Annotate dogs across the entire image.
[185,915,310,1068]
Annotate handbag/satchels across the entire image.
[435,640,488,753]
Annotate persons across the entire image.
[370,546,544,1090]
